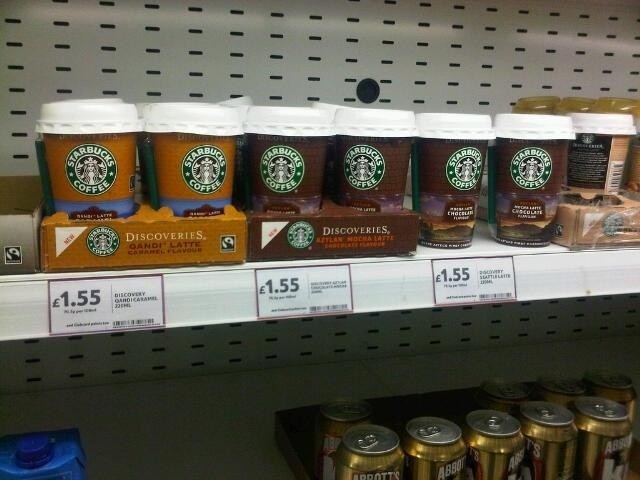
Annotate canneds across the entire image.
[462,408,526,480]
[404,416,467,479]
[317,397,375,480]
[335,423,404,480]
[479,374,640,479]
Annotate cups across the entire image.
[241,104,335,215]
[489,112,577,248]
[33,96,145,222]
[409,111,497,252]
[141,100,246,219]
[564,112,638,208]
[331,107,416,214]
